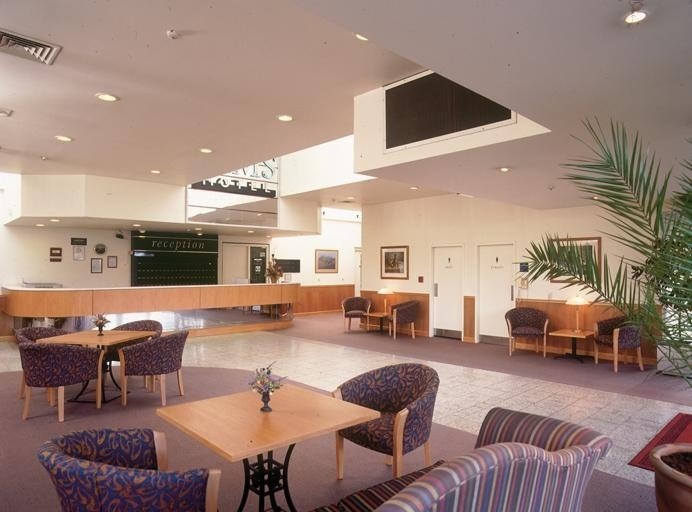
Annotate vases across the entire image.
[260,391,272,412]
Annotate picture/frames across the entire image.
[380,245,409,280]
[73,246,85,261]
[91,258,103,273]
[107,255,118,268]
[549,236,602,283]
[315,249,339,274]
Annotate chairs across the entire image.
[341,296,371,333]
[332,363,440,480]
[15,319,189,422]
[505,307,550,358]
[593,314,644,373]
[389,299,417,340]
[37,427,222,512]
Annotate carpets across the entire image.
[0,366,655,512]
[268,309,692,406]
[627,412,692,472]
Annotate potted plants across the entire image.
[510,114,692,512]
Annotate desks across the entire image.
[155,384,381,512]
[549,328,594,363]
[369,311,393,336]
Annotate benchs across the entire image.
[307,407,613,512]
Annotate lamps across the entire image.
[377,287,394,314]
[565,296,590,335]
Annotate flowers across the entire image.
[248,361,289,396]
